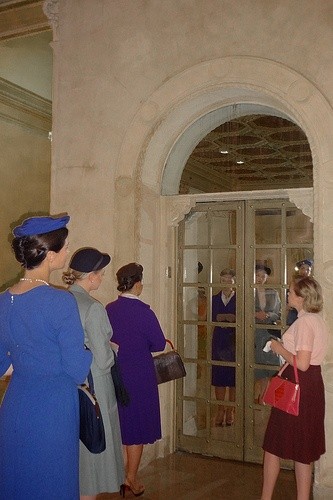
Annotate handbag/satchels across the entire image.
[267,311,281,338]
[152,340,186,385]
[110,349,127,407]
[77,344,107,454]
[263,355,300,416]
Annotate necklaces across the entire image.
[19,278,50,287]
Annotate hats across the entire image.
[296,260,312,269]
[69,248,110,273]
[220,268,236,276]
[12,216,70,238]
[256,264,271,276]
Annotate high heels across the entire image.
[119,484,146,498]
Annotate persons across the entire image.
[286,259,312,331]
[62,249,126,500]
[105,262,166,499]
[253,265,282,407]
[212,268,236,426]
[260,275,327,500]
[197,282,207,380]
[0,216,94,500]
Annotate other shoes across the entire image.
[215,409,225,425]
[226,408,233,426]
